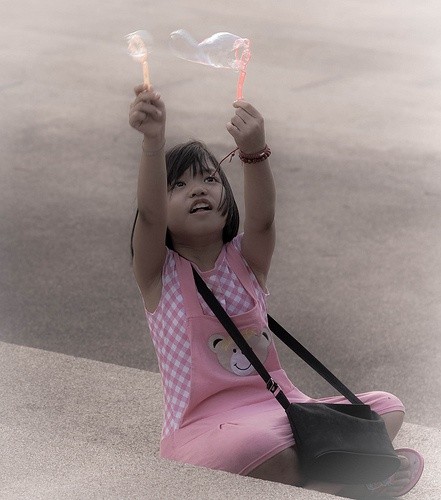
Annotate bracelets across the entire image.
[209,145,271,178]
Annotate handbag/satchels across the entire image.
[282,401,396,483]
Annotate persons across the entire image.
[129,84,424,500]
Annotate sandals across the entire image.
[348,447,425,498]
